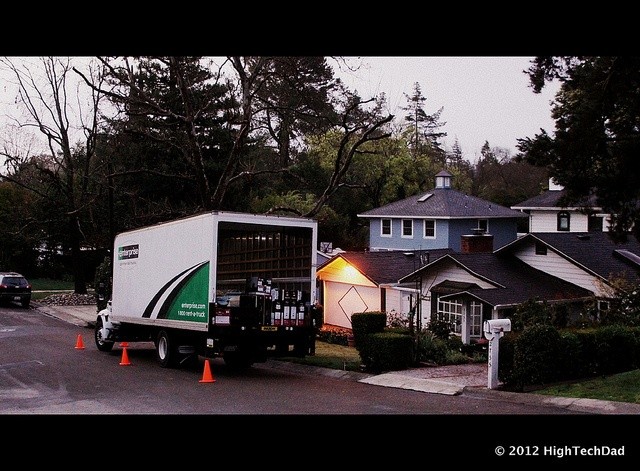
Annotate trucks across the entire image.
[94,210,319,368]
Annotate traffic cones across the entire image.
[74,334,85,350]
[198,360,216,384]
[119,342,129,347]
[118,347,132,367]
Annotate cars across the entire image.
[0,272,31,308]
